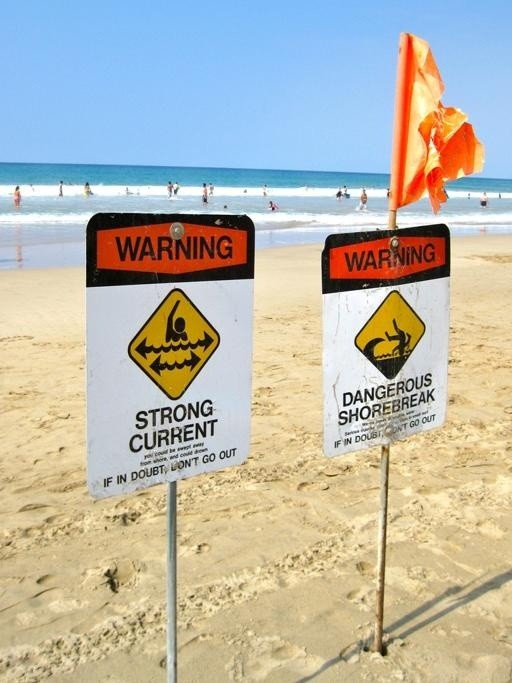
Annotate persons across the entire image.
[167,182,179,199]
[201,183,279,210]
[58,180,92,197]
[335,185,390,209]
[445,188,501,208]
[13,186,21,206]
